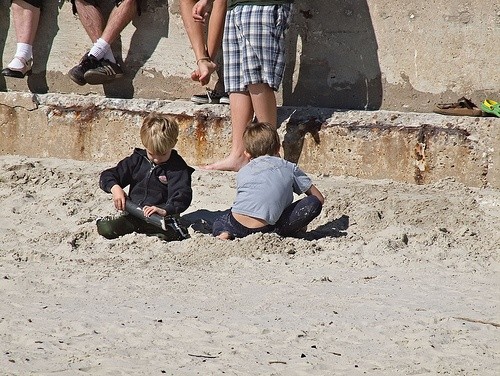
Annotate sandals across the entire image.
[480,99,500,117]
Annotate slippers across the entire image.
[434,97,482,116]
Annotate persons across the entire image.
[192,0,296,172]
[180,1,226,87]
[96,115,195,242]
[68,0,144,85]
[213,122,324,240]
[0,0,42,78]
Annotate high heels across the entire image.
[1,56,33,78]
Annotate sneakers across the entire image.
[68,51,99,86]
[84,59,123,85]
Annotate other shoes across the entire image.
[191,87,220,104]
[220,92,230,104]
